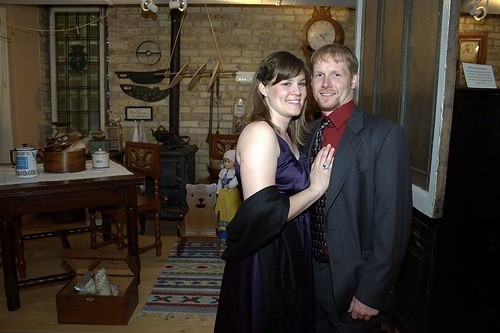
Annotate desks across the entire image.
[0,157,145,311]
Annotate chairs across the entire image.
[97,141,162,256]
[177,183,221,256]
[199,134,239,184]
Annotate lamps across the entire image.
[169,0,187,11]
[140,0,158,13]
[470,0,489,21]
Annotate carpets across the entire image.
[143,236,227,322]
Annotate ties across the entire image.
[308,118,331,259]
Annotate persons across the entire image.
[301,44,413,333]
[213,51,334,333]
[215,150,241,239]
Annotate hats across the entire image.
[223,150,235,163]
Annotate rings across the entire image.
[323,163,328,170]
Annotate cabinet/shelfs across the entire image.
[105,123,123,153]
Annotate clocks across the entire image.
[301,6,347,122]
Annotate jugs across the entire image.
[9,143,39,178]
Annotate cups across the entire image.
[91,148,110,168]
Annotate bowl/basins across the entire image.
[43,147,86,174]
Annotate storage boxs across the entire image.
[55,249,138,327]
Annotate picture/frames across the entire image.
[457,32,488,65]
[125,106,153,121]
